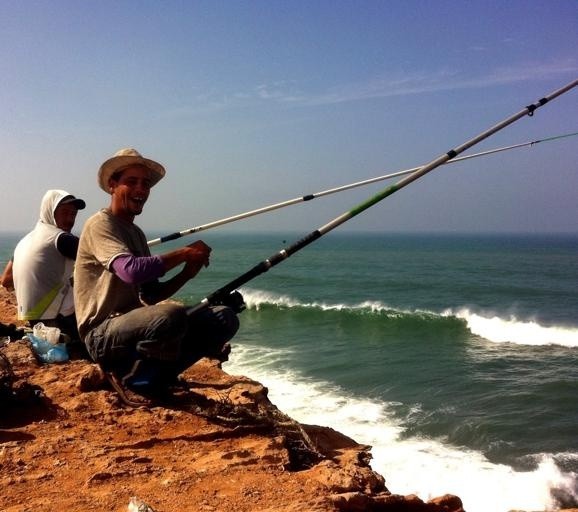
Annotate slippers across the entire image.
[106,368,189,408]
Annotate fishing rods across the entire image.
[145,134,577,245]
[187,76,578,316]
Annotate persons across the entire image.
[73,148,240,408]
[0,189,86,342]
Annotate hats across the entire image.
[58,196,86,210]
[96,148,167,195]
